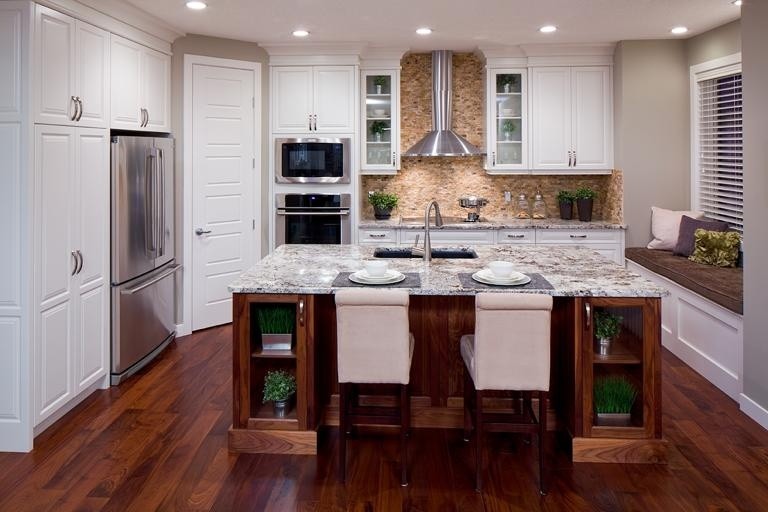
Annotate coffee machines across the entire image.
[458,197,488,222]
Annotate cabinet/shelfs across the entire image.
[226,292,332,455]
[496,230,535,246]
[554,298,661,463]
[1,122,110,453]
[358,67,401,176]
[534,230,627,267]
[527,65,614,177]
[0,0,108,130]
[271,65,356,136]
[483,68,529,177]
[108,30,171,138]
[356,230,396,247]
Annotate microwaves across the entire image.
[275,137,351,184]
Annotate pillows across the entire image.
[671,214,727,259]
[646,205,703,252]
[688,228,739,270]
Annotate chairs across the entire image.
[333,288,416,489]
[458,291,553,497]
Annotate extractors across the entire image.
[401,51,484,158]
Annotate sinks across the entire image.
[372,246,480,260]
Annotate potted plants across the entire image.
[373,77,385,94]
[593,312,625,356]
[496,75,517,93]
[367,191,399,220]
[592,374,638,427]
[257,309,293,353]
[500,120,515,141]
[260,369,296,417]
[553,189,576,220]
[368,121,386,141]
[574,186,598,221]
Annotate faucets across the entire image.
[422,199,443,263]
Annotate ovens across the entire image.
[276,193,352,249]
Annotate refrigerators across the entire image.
[110,136,184,385]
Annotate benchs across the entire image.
[623,246,744,407]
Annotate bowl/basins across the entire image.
[374,110,385,115]
[363,260,388,276]
[503,109,513,114]
[489,260,514,277]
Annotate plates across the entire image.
[497,113,519,117]
[354,269,401,282]
[367,115,390,118]
[476,270,526,285]
[348,270,405,284]
[471,270,532,286]
